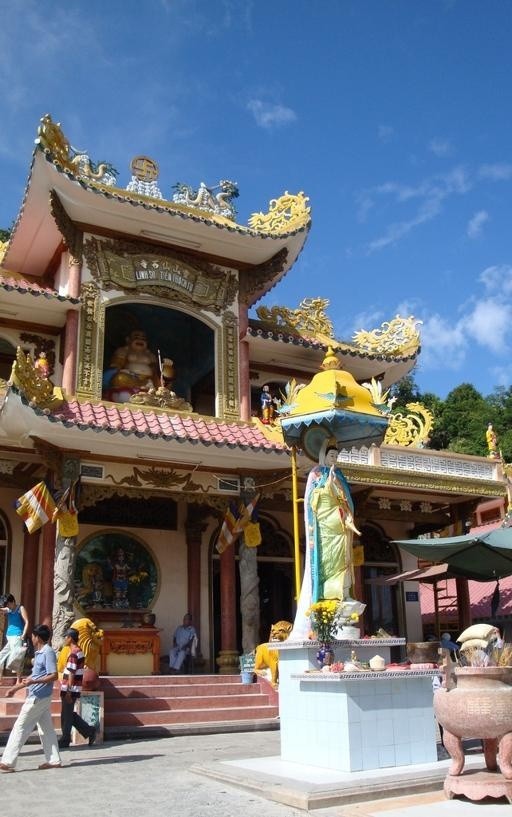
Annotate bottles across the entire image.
[350,650,358,661]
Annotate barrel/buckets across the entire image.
[239,670,255,684]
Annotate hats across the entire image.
[62,628,79,638]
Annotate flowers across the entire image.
[304,600,359,643]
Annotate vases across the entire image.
[240,672,254,683]
[316,642,334,669]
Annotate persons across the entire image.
[106,548,129,597]
[57,627,97,748]
[304,434,362,602]
[35,351,49,377]
[167,613,197,674]
[102,328,177,402]
[0,592,31,684]
[260,385,274,424]
[0,624,62,772]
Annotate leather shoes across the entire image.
[0,764,15,773]
[38,764,61,770]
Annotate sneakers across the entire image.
[58,741,70,748]
[88,727,97,745]
[169,668,176,674]
